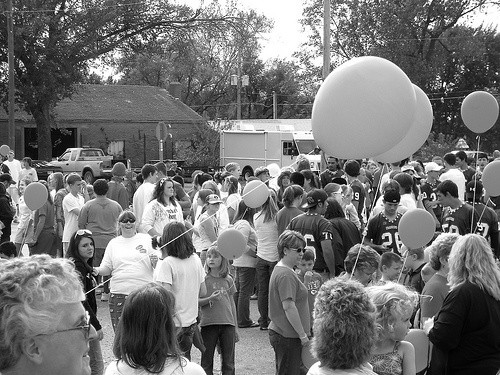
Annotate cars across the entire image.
[30,160,63,180]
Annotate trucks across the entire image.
[218,130,322,181]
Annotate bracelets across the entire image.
[299,334,307,340]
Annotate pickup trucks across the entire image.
[44,148,131,185]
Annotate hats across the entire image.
[301,189,328,208]
[205,194,224,204]
[425,162,443,174]
[343,160,360,175]
[254,166,269,177]
[0,173,16,184]
[384,190,400,203]
[466,180,483,199]
[111,162,126,176]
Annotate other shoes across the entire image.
[95,290,101,294]
[249,321,259,327]
[260,327,268,330]
[250,294,258,300]
[101,292,110,300]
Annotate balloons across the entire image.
[0,144,10,157]
[398,208,437,251]
[376,82,433,163]
[243,180,269,208]
[461,91,500,134]
[311,56,417,160]
[217,229,247,260]
[24,182,48,212]
[482,160,500,197]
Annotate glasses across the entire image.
[119,218,135,223]
[290,248,305,253]
[33,311,90,339]
[331,191,343,196]
[75,230,92,239]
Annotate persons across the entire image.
[0,148,500,375]
